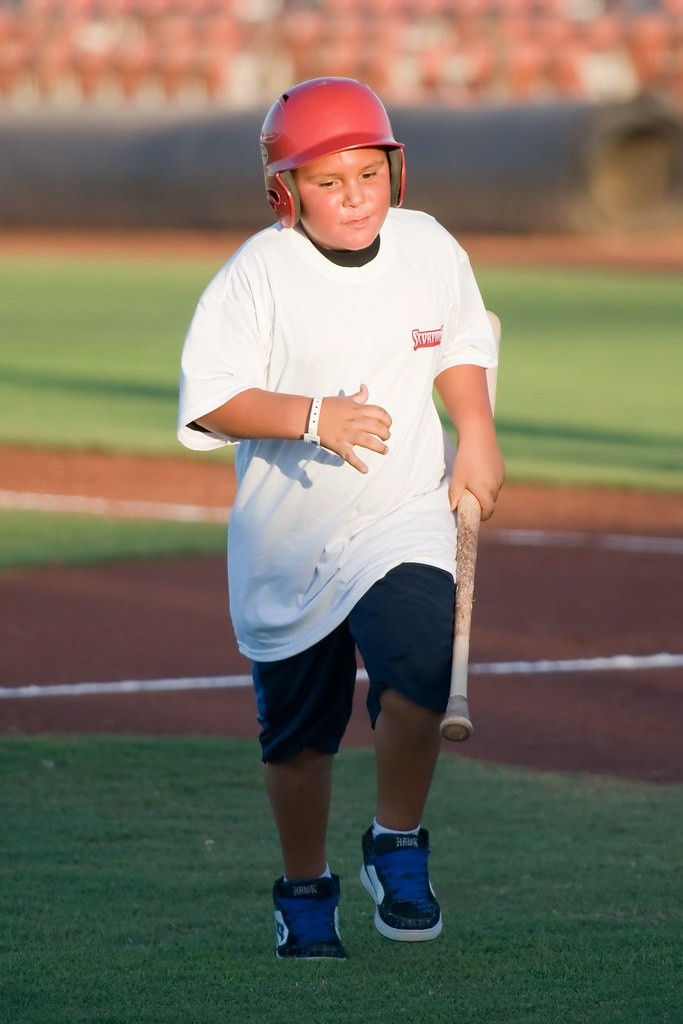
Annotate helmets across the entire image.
[259,77,406,228]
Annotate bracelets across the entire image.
[304,395,324,446]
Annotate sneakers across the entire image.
[360,825,442,940]
[272,872,348,961]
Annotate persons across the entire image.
[176,75,506,964]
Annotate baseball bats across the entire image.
[440,310,500,741]
